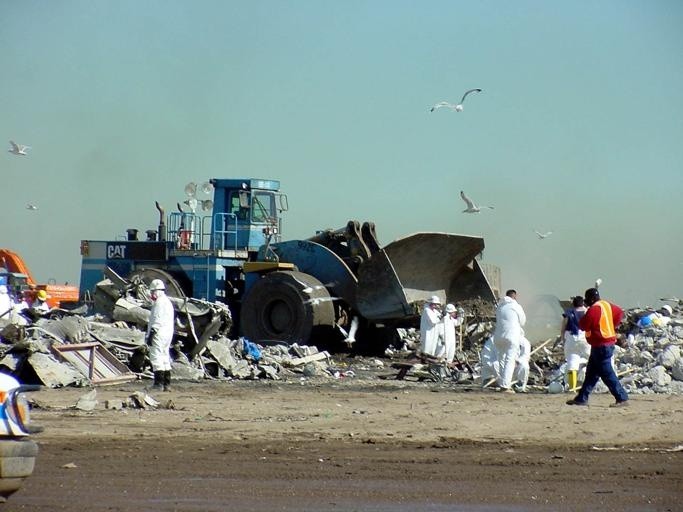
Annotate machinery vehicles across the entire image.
[80,178,498,349]
[1,247,81,310]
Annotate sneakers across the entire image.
[610,400,629,407]
[566,400,588,406]
[483,377,528,393]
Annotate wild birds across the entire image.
[26,203,38,213]
[5,138,31,156]
[335,314,359,350]
[429,86,483,113]
[659,296,680,304]
[534,229,554,241]
[460,190,494,215]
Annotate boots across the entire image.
[146,371,171,392]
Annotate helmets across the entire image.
[150,280,166,291]
[661,305,672,315]
[38,290,47,300]
[445,304,458,313]
[586,288,598,300]
[428,296,441,304]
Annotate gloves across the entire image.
[147,328,155,346]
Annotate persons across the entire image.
[419,287,631,408]
[0,285,50,325]
[143,279,175,392]
